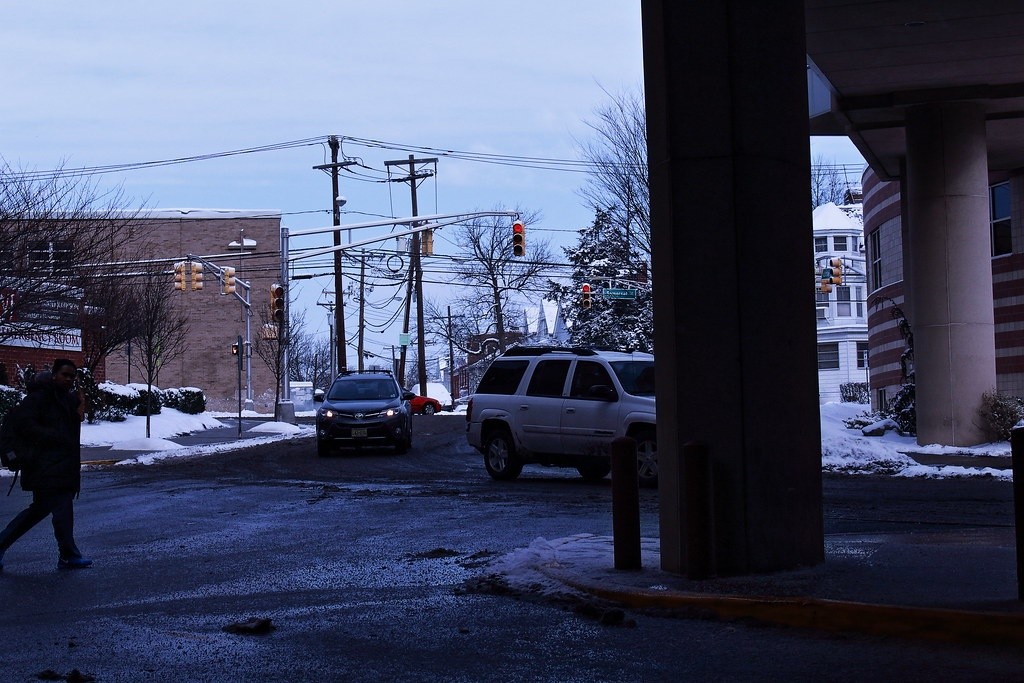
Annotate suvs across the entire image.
[311,369,416,458]
[410,382,454,411]
[463,346,657,493]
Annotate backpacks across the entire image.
[0,382,56,471]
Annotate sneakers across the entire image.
[57,556,95,569]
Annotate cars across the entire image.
[402,387,442,416]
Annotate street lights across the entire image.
[332,194,348,376]
[358,296,404,375]
[412,250,465,398]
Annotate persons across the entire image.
[0,358,93,570]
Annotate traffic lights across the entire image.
[820,268,832,293]
[173,261,187,292]
[231,344,243,355]
[224,267,236,294]
[191,261,204,291]
[270,284,285,322]
[829,258,843,285]
[582,282,591,309]
[512,221,526,256]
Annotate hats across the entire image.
[52,358,77,375]
